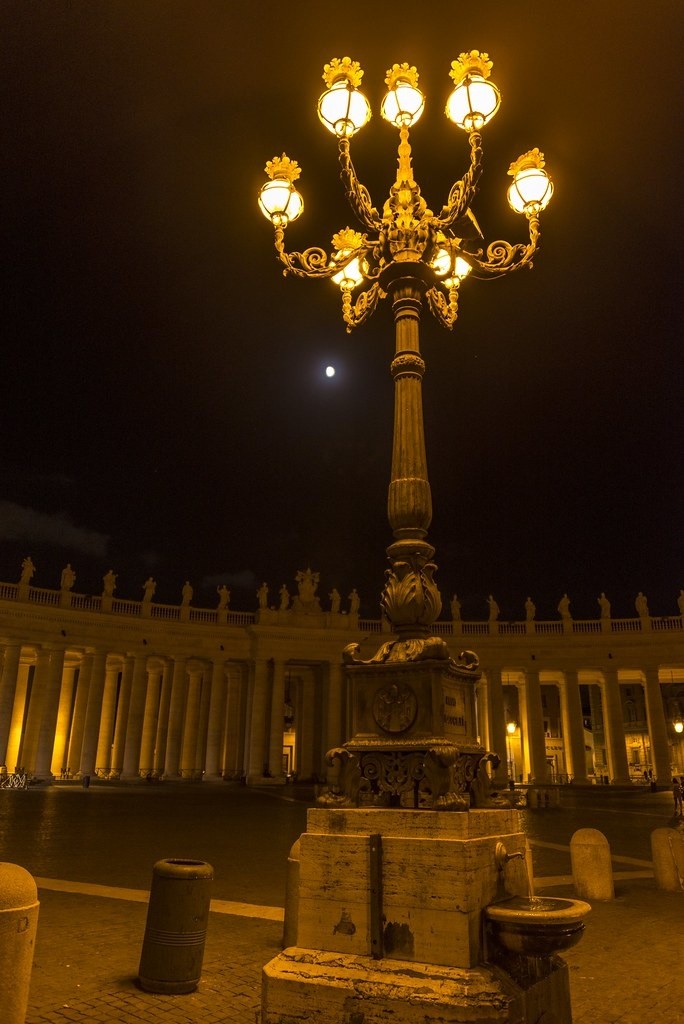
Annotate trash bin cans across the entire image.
[138,858,214,994]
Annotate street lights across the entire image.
[258,51,557,809]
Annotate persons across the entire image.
[14,555,36,601]
[643,769,648,780]
[141,575,157,618]
[328,587,342,614]
[648,768,654,779]
[277,583,291,612]
[180,581,194,624]
[672,778,682,817]
[486,593,501,634]
[59,563,77,611]
[557,592,574,632]
[449,593,464,635]
[101,568,119,614]
[650,780,653,789]
[680,776,684,801]
[215,584,232,626]
[634,591,654,633]
[347,588,361,619]
[255,581,269,615]
[524,596,537,636]
[293,564,322,614]
[597,591,613,632]
